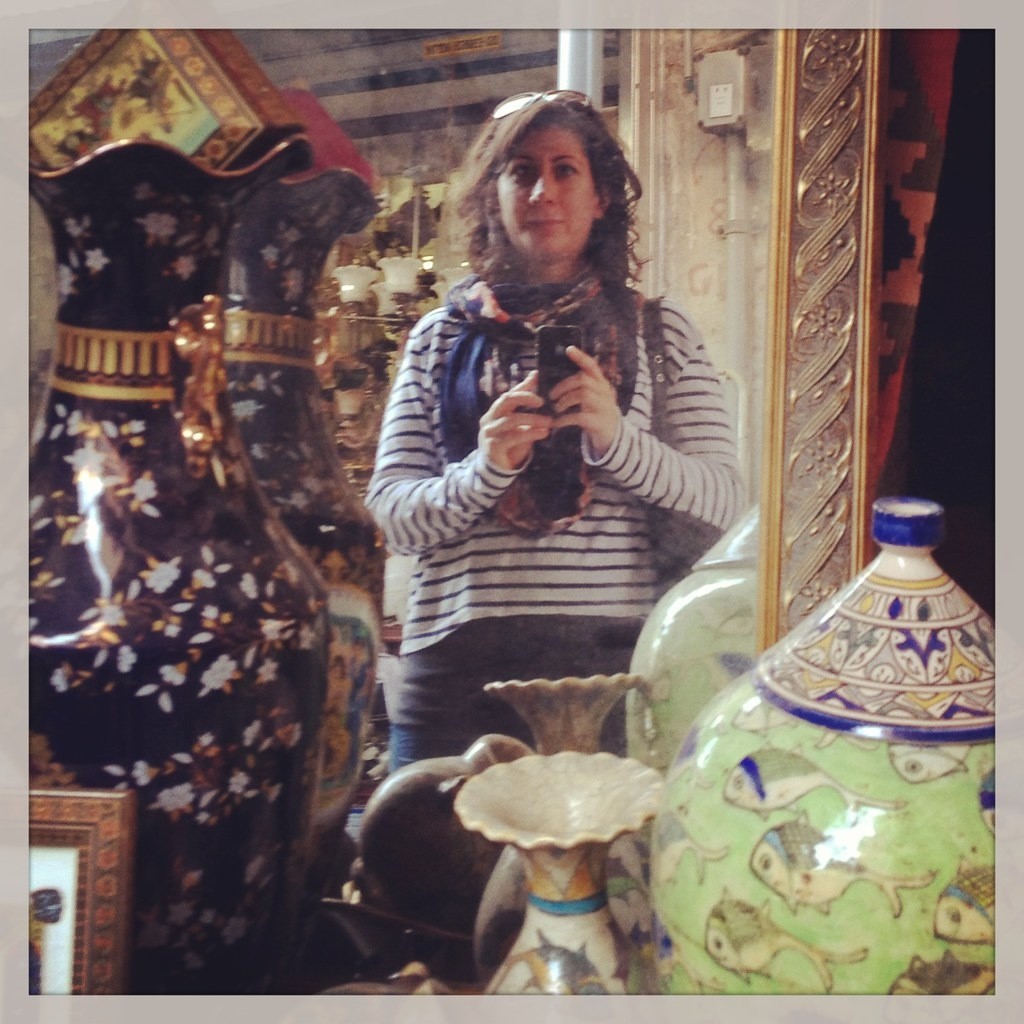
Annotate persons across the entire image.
[362,90,743,774]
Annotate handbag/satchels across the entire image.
[642,296,724,591]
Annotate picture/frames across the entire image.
[29,791,134,995]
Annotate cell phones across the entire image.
[534,324,586,417]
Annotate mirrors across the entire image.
[29,29,880,995]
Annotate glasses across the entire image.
[493,87,591,118]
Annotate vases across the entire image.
[453,749,667,994]
[29,29,329,995]
[225,89,377,995]
[650,499,995,995]
[622,499,760,778]
[475,672,665,995]
